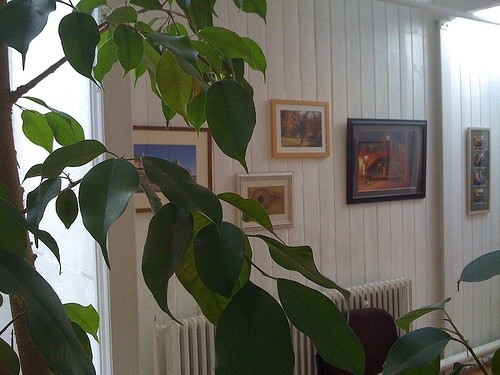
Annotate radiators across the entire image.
[164,276,414,375]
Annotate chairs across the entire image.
[315,308,399,375]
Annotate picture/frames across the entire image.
[346,118,428,205]
[269,99,330,159]
[132,125,213,213]
[467,126,492,216]
[235,172,296,232]
